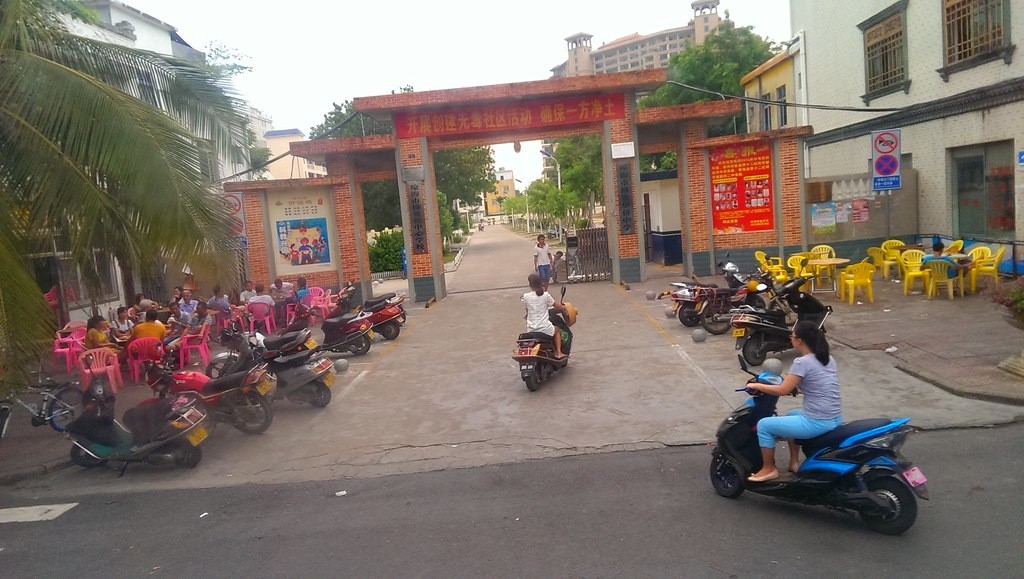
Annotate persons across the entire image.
[919,242,968,296]
[480,218,495,230]
[747,321,845,481]
[523,274,568,358]
[85,278,311,384]
[533,234,565,292]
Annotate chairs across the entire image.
[754,240,1006,305]
[44,282,338,394]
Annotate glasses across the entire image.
[789,335,800,339]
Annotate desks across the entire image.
[190,310,221,350]
[158,307,170,324]
[112,330,171,347]
[922,253,968,265]
[807,258,851,298]
[888,246,928,275]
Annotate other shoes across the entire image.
[748,469,780,482]
[787,462,799,474]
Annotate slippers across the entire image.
[557,353,568,358]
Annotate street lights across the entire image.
[515,178,530,233]
[540,150,562,243]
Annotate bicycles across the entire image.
[0,379,85,444]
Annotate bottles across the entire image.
[933,234,940,245]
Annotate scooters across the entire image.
[547,224,567,239]
[63,352,210,478]
[709,354,930,537]
[205,280,407,406]
[668,249,835,366]
[510,286,574,393]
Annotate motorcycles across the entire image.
[143,349,278,435]
